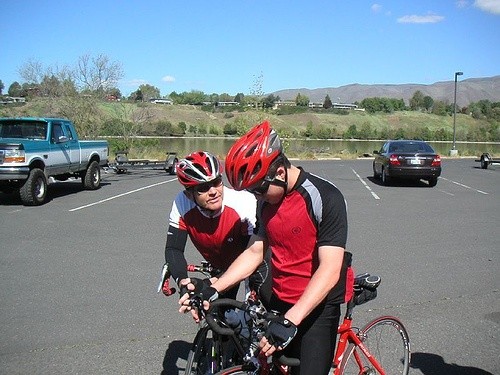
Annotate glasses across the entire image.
[246,179,270,196]
[194,178,222,192]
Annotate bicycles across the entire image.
[157,260,411,375]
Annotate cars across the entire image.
[373,140,442,188]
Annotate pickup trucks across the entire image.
[0,117,109,206]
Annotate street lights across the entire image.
[449,72,464,157]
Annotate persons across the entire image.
[190,120,354,375]
[166,151,255,339]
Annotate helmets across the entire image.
[176,151,223,188]
[225,120,282,191]
